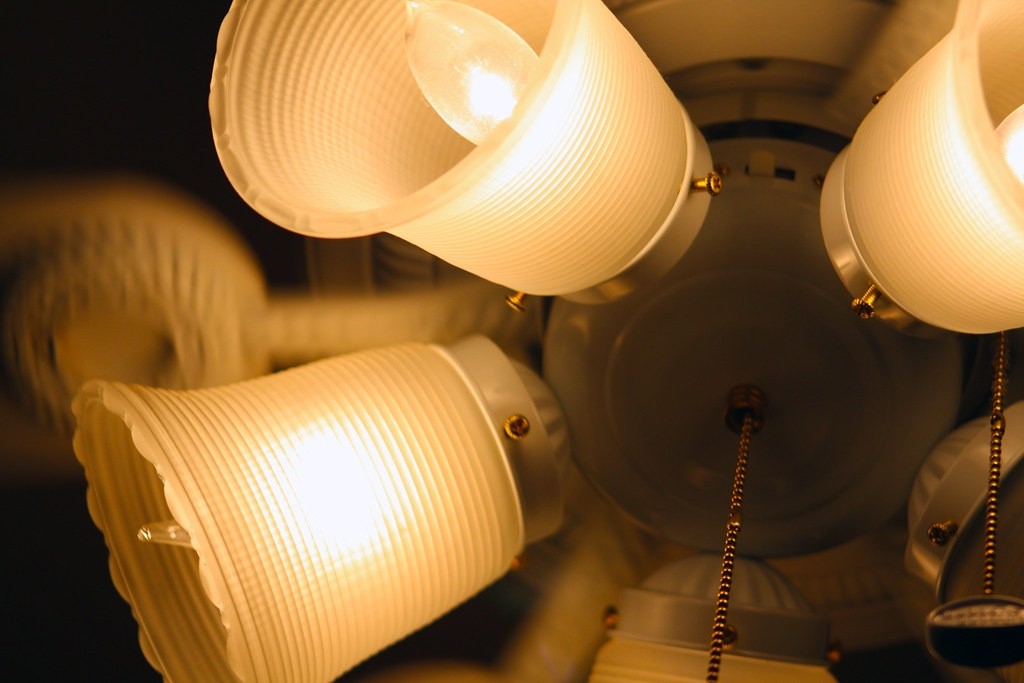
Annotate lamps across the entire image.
[65,0,1024,683]
